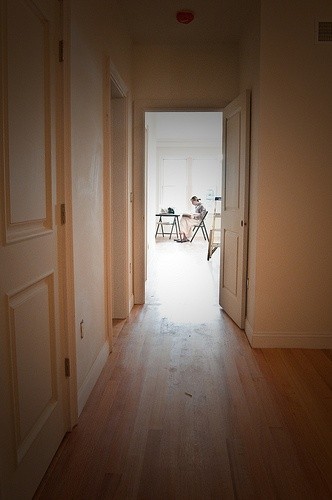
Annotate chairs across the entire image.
[190,211,209,242]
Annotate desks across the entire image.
[155,213,180,240]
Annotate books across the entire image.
[182,214,190,217]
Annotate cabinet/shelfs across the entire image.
[206,197,223,262]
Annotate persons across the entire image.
[174,196,206,242]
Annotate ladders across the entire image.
[207,196,222,262]
